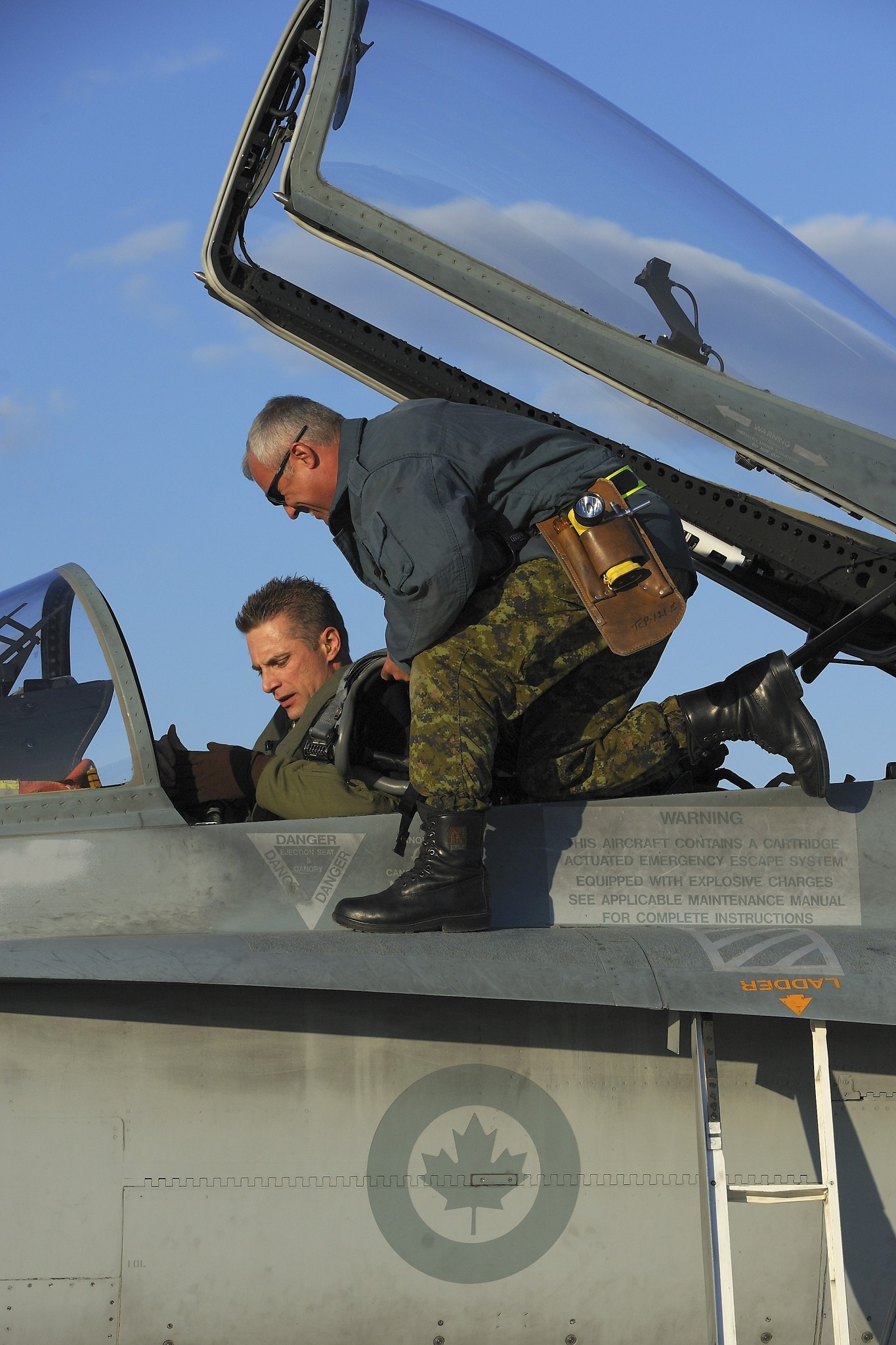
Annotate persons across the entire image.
[155,575,410,823]
[241,394,834,935]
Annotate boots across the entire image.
[332,798,491,932]
[673,648,831,797]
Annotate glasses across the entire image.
[264,424,308,506]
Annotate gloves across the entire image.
[170,741,268,805]
[154,722,188,788]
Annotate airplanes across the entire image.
[3,1,895,1339]
